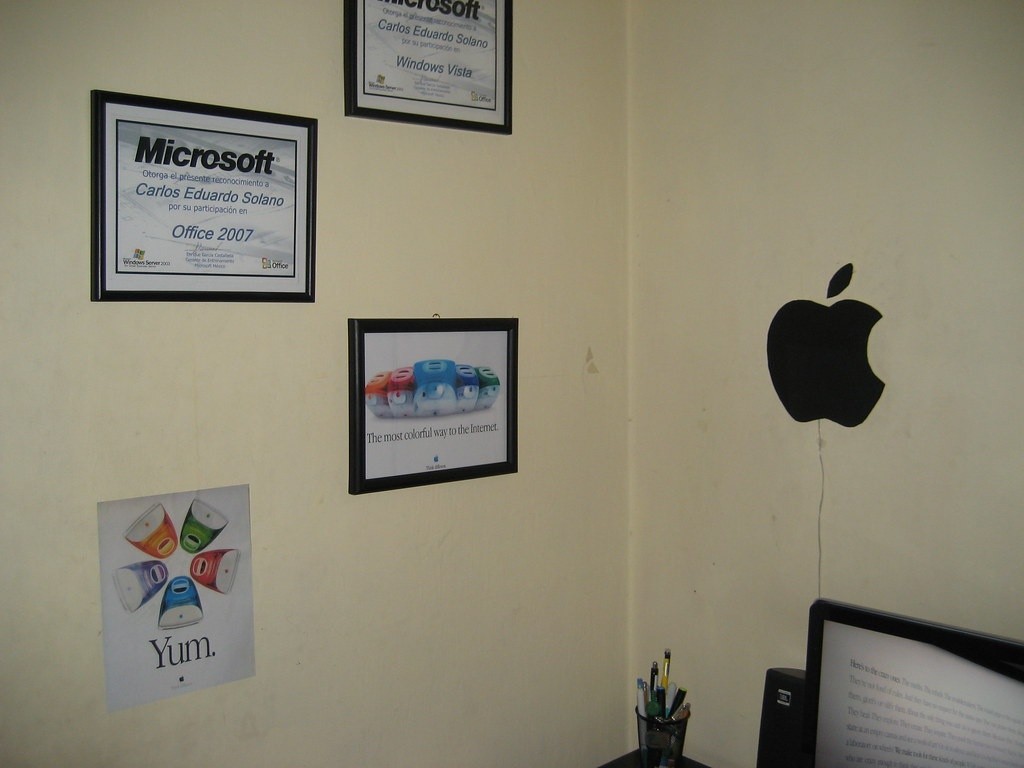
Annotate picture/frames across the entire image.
[347,316,518,496]
[91,89,319,303]
[343,0,513,135]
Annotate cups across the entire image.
[635,705,690,768]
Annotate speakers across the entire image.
[756,667,805,768]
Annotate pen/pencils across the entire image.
[636,648,692,768]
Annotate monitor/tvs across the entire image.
[798,596,1024,768]
